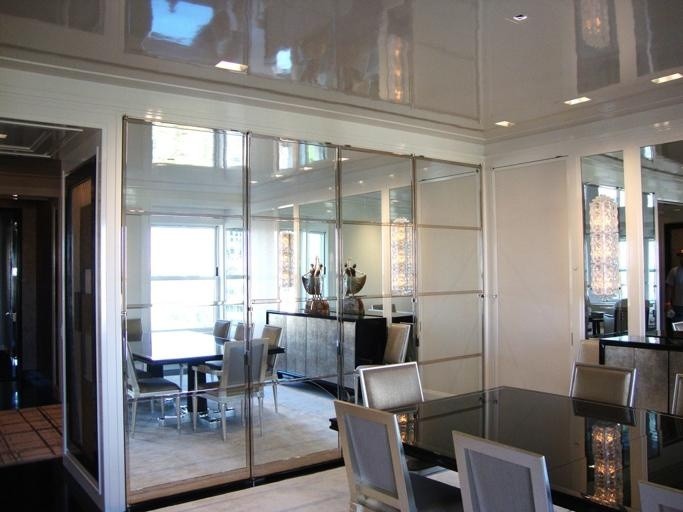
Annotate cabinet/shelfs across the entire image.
[598,337,682,414]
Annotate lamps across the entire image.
[275,227,296,291]
[388,211,417,297]
[590,421,625,507]
[586,193,620,301]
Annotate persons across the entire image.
[665,251,682,317]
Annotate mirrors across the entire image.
[639,139,682,337]
[579,150,627,342]
[121,115,488,506]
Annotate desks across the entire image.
[328,385,682,512]
[263,310,386,402]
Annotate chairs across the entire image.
[671,373,682,418]
[120,304,285,443]
[451,430,554,512]
[334,398,462,512]
[360,361,451,478]
[383,321,412,364]
[568,362,637,408]
[586,294,627,338]
